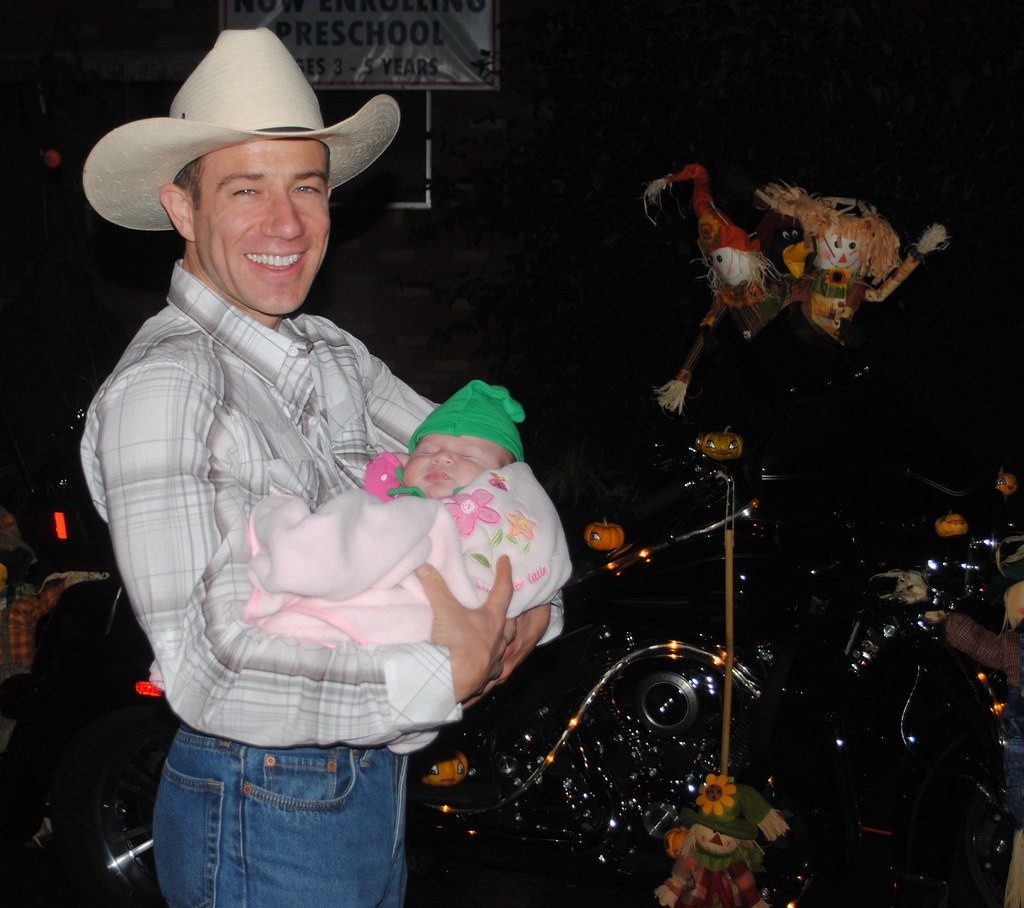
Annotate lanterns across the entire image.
[934,513,967,537]
[996,472,1017,496]
[584,518,625,549]
[699,427,743,459]
[422,750,468,786]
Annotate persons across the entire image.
[649,164,947,415]
[940,545,1024,907]
[249,379,573,644]
[651,774,789,908]
[80,26,564,908]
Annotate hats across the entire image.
[408,380,525,463]
[82,26,401,229]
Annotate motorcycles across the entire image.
[1,187,1024,908]
